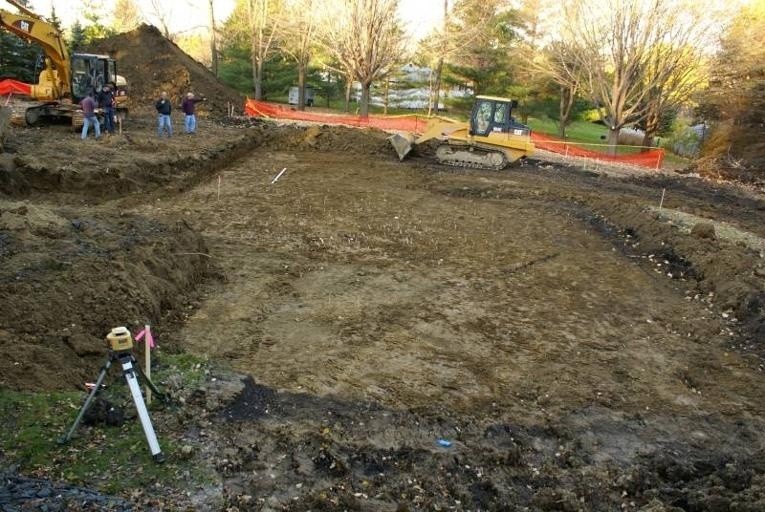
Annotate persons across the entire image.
[179,91,208,135]
[154,91,174,139]
[80,92,101,141]
[98,83,119,134]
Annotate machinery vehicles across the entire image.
[0,0,130,128]
[389,87,534,171]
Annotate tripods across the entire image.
[63,351,169,464]
